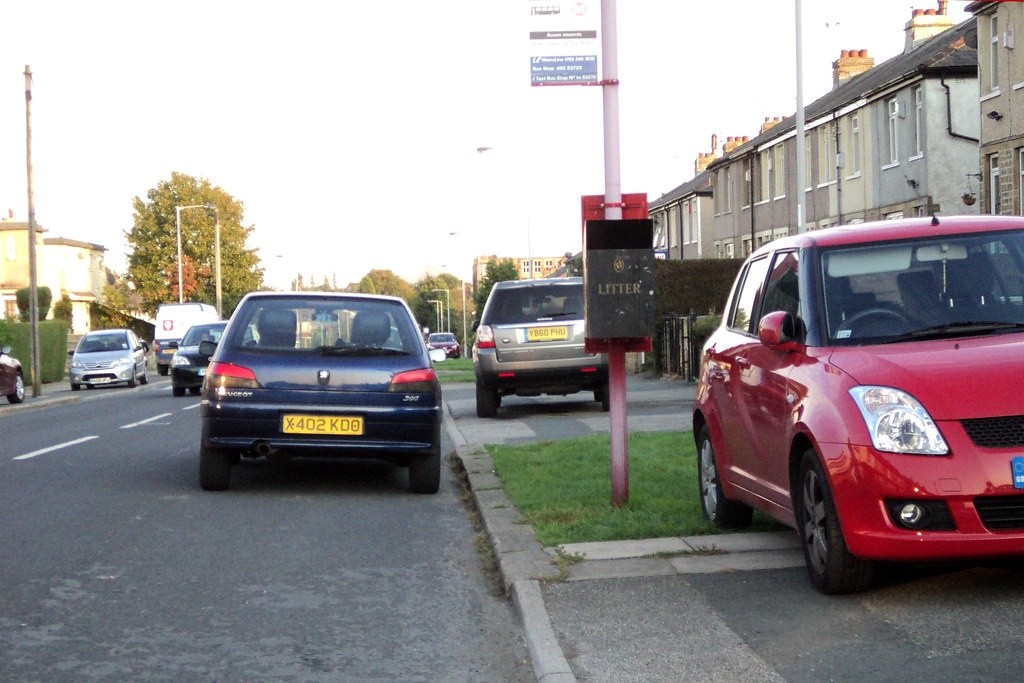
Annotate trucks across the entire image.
[153,301,222,377]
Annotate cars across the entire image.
[426,332,459,358]
[0,344,26,405]
[166,321,253,396]
[65,328,152,391]
[693,210,1024,594]
[198,290,441,497]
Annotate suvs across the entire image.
[471,279,619,418]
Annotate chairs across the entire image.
[253,305,394,349]
[826,248,1006,333]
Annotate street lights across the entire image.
[424,287,451,332]
[175,205,219,305]
